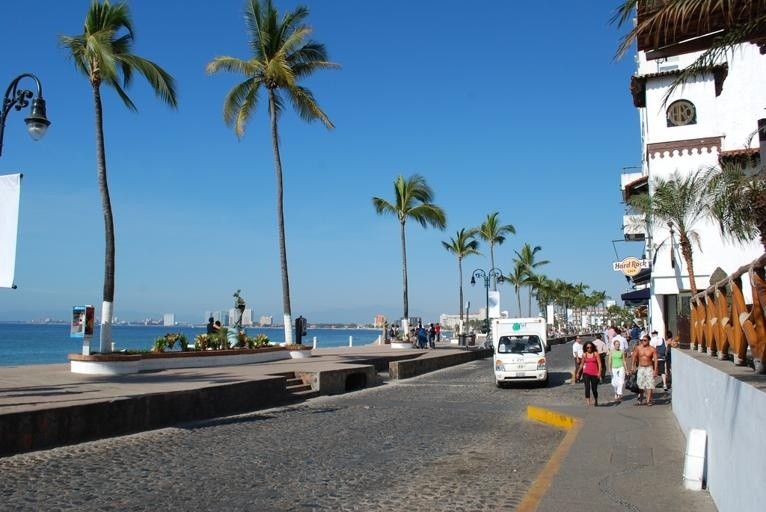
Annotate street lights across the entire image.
[471,268,505,349]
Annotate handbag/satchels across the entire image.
[625,370,639,393]
[656,343,666,356]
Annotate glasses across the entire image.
[642,339,648,342]
[585,347,593,349]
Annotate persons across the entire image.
[435,323,441,342]
[390,324,400,337]
[207,317,223,333]
[528,336,540,349]
[408,321,436,349]
[572,324,672,407]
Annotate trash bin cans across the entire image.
[457,334,475,345]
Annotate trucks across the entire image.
[489,315,550,389]
